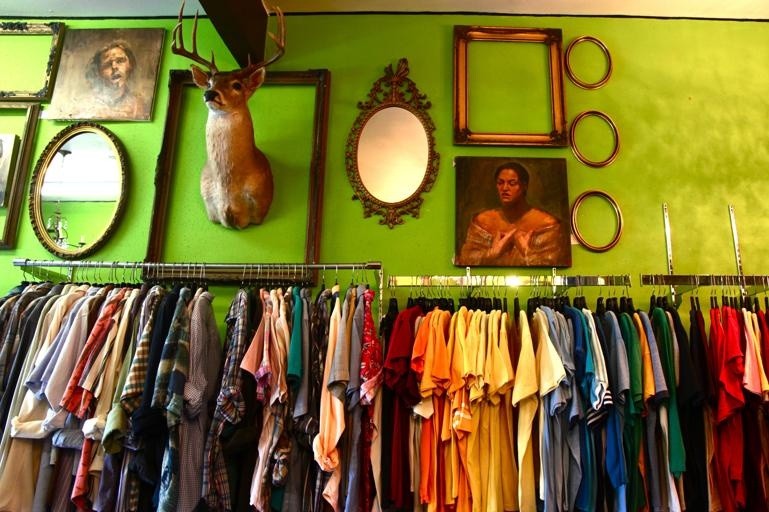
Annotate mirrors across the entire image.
[343,56,441,232]
[26,122,133,262]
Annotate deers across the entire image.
[171,0,286,230]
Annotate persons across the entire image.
[458,161,567,264]
[81,37,148,120]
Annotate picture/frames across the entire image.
[451,23,570,148]
[0,21,66,250]
[139,68,332,287]
